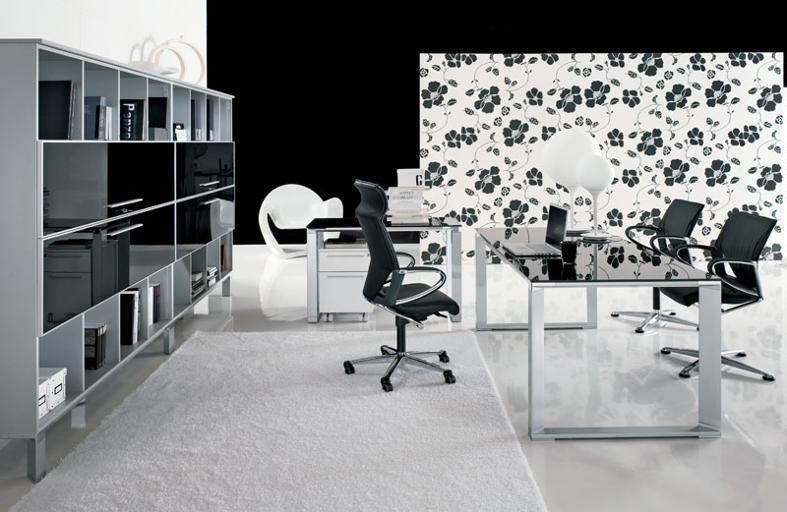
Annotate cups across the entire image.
[560,240,578,266]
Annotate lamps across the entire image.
[579,155,613,240]
[539,127,598,233]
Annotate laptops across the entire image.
[502,205,568,258]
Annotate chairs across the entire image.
[661,213,777,381]
[611,199,705,333]
[343,180,460,392]
[258,184,344,260]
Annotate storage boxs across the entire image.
[38,384,50,419]
[39,368,66,412]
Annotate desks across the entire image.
[307,218,462,323]
[476,228,721,440]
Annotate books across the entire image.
[83,240,227,373]
[387,185,429,224]
[38,78,211,143]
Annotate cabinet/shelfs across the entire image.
[0,39,235,485]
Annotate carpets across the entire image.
[7,332,548,512]
